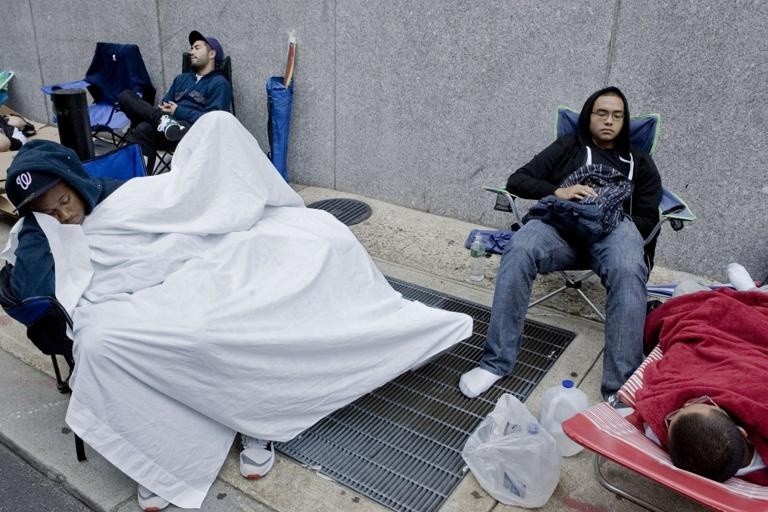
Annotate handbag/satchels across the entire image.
[465,229,515,254]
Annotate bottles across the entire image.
[470,236,485,282]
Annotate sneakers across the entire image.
[158,115,188,141]
[136,482,171,511]
[239,433,275,480]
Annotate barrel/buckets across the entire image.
[539,380,590,458]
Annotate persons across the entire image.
[116,31,233,159]
[457,86,663,421]
[5,140,275,511]
[642,261,768,484]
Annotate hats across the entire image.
[188,30,224,63]
[9,171,62,213]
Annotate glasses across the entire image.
[591,110,624,121]
[664,395,729,429]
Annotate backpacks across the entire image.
[526,163,634,243]
